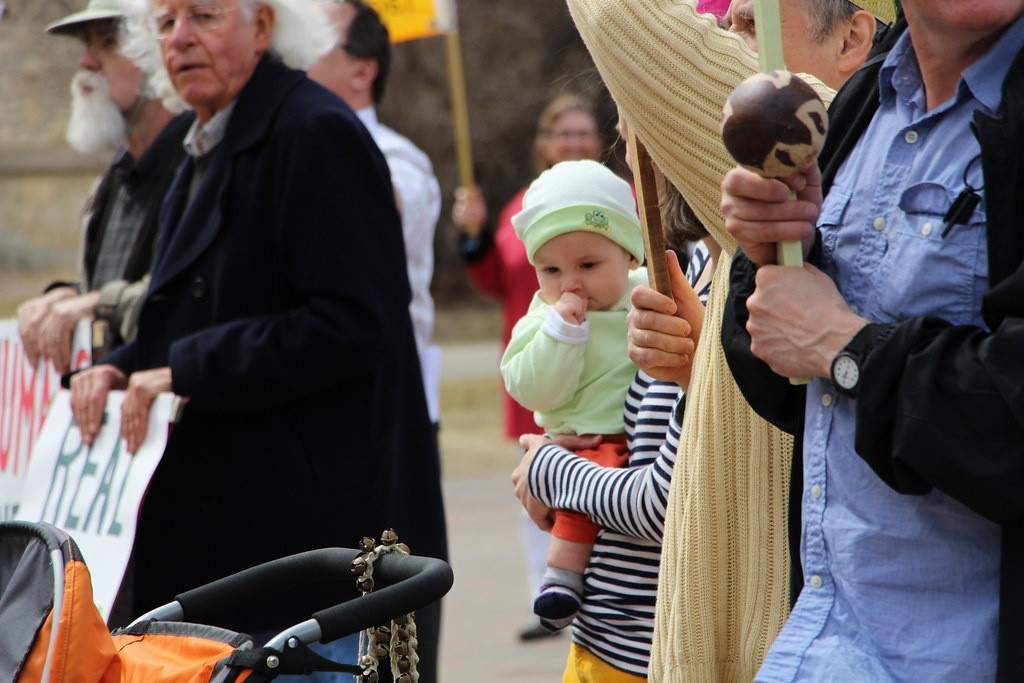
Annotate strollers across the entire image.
[0,517,454,683]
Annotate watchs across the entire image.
[830,319,886,398]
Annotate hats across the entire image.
[511,160,644,270]
[848,0,898,29]
[47,0,133,35]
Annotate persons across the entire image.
[16,0,197,378]
[302,0,444,683]
[446,92,612,639]
[68,0,451,682]
[714,0,1024,683]
[511,180,722,683]
[566,0,899,683]
[498,158,651,635]
